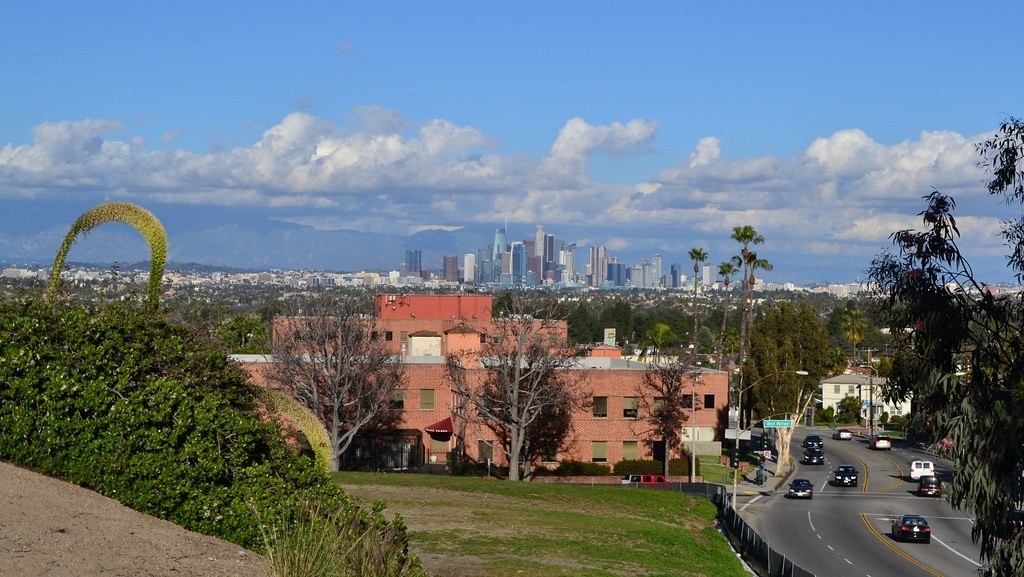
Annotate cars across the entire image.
[869,434,892,451]
[891,514,931,543]
[802,446,825,465]
[803,435,824,448]
[832,429,852,440]
[833,464,859,487]
[788,479,814,499]
[917,475,942,497]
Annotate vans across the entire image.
[908,459,936,481]
[620,473,669,487]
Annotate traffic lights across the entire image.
[761,433,768,449]
[730,447,740,468]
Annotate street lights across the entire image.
[859,364,879,434]
[732,369,809,512]
[690,375,703,483]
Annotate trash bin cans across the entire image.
[754,473,767,484]
